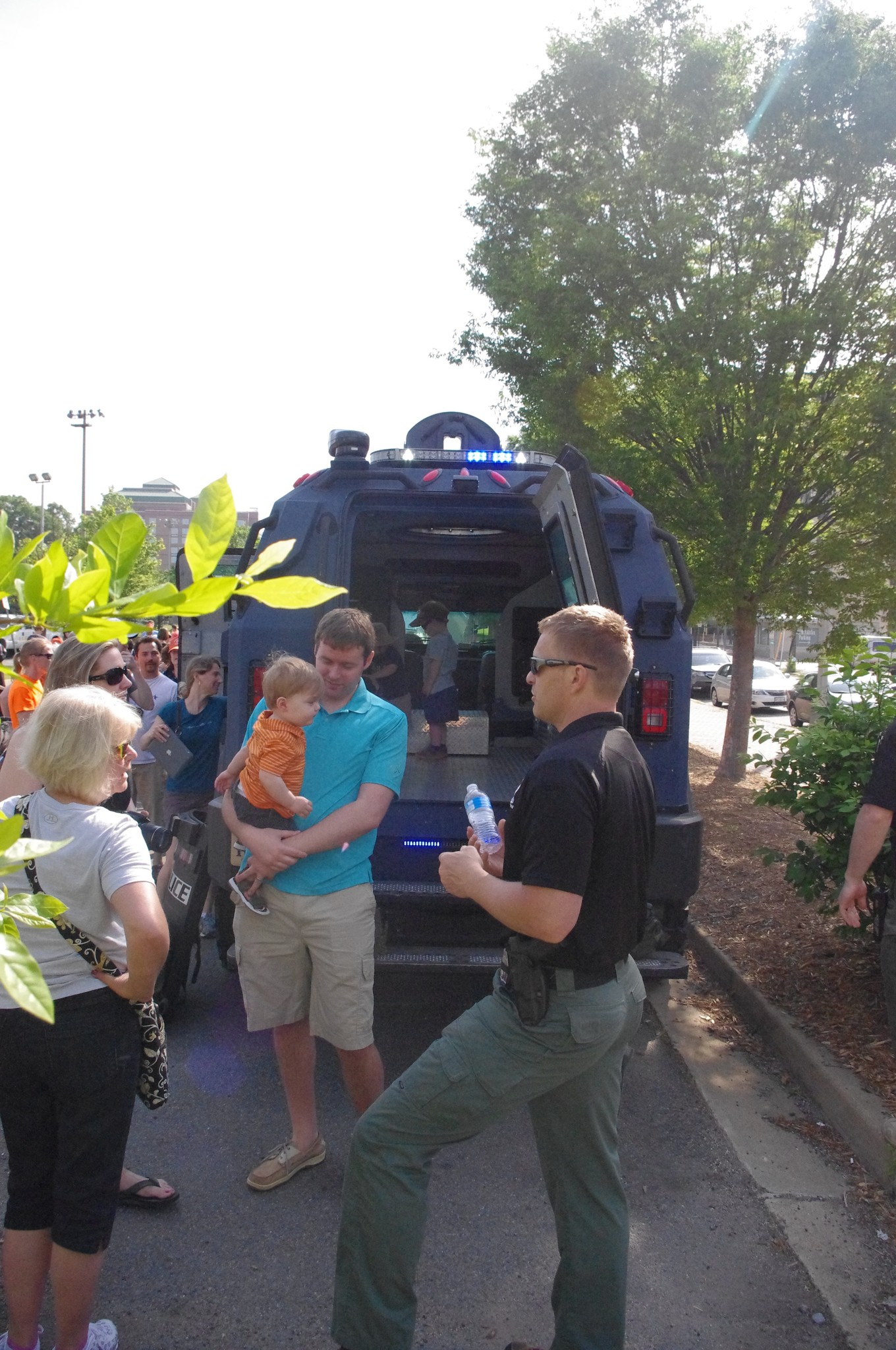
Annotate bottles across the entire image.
[464,783,503,855]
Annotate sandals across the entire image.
[229,877,270,915]
[233,838,247,851]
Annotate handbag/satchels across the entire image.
[128,996,169,1109]
[145,724,193,780]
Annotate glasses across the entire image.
[28,653,54,660]
[529,656,598,676]
[88,664,128,687]
[115,740,133,759]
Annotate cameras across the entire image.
[115,809,173,854]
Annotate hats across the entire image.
[410,601,448,627]
[371,622,395,647]
[168,636,179,652]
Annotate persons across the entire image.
[222,608,408,1191]
[0,638,179,1205]
[837,717,896,1054]
[331,607,654,1350]
[409,601,459,761]
[361,622,410,722]
[215,653,326,915]
[0,621,229,936]
[0,688,171,1350]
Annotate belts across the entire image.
[543,953,630,992]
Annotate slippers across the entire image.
[118,1174,179,1206]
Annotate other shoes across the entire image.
[506,1340,541,1350]
[415,742,448,759]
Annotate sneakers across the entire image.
[882,1118,896,1146]
[52,1318,118,1350]
[247,1132,326,1189]
[0,1324,44,1350]
